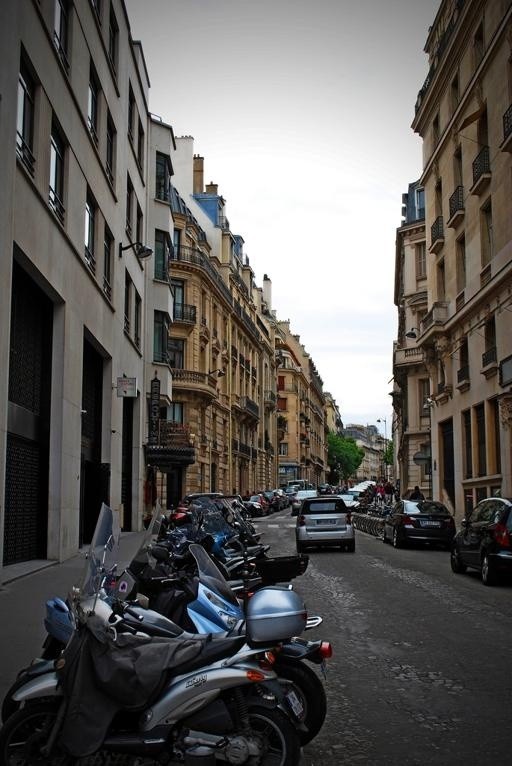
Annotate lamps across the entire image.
[209,369,226,377]
[405,327,420,339]
[119,241,153,258]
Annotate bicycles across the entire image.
[352,506,391,538]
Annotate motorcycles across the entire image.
[0,499,330,748]
[1,502,308,765]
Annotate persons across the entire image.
[373,480,399,508]
[408,486,425,500]
[325,484,350,495]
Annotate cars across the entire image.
[185,479,306,516]
[317,480,376,512]
[292,490,322,516]
[382,500,456,548]
[450,498,512,586]
[295,497,355,553]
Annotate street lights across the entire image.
[376,416,387,478]
